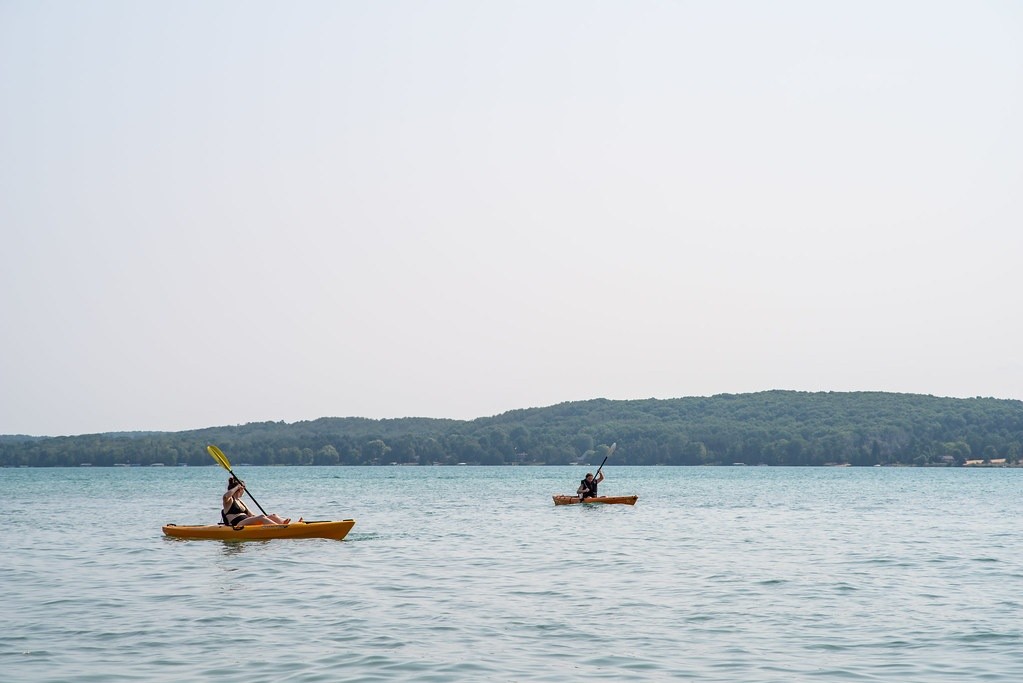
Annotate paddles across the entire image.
[206,444,268,516]
[580,442,617,504]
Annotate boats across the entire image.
[161,521,354,540]
[551,494,638,504]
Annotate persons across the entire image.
[577,469,608,498]
[223,477,292,526]
